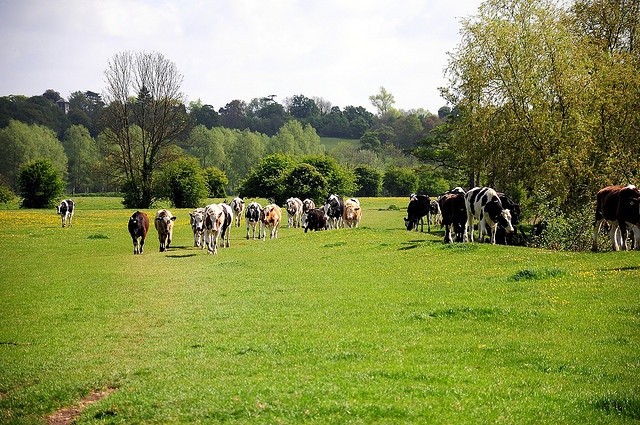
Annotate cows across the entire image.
[127,211,150,256]
[259,202,282,241]
[201,201,227,252]
[154,209,177,252]
[591,184,640,252]
[430,185,465,246]
[244,201,262,241]
[189,207,207,248]
[230,196,245,228]
[462,186,515,244]
[403,193,431,233]
[282,193,363,233]
[485,188,521,245]
[55,198,75,228]
